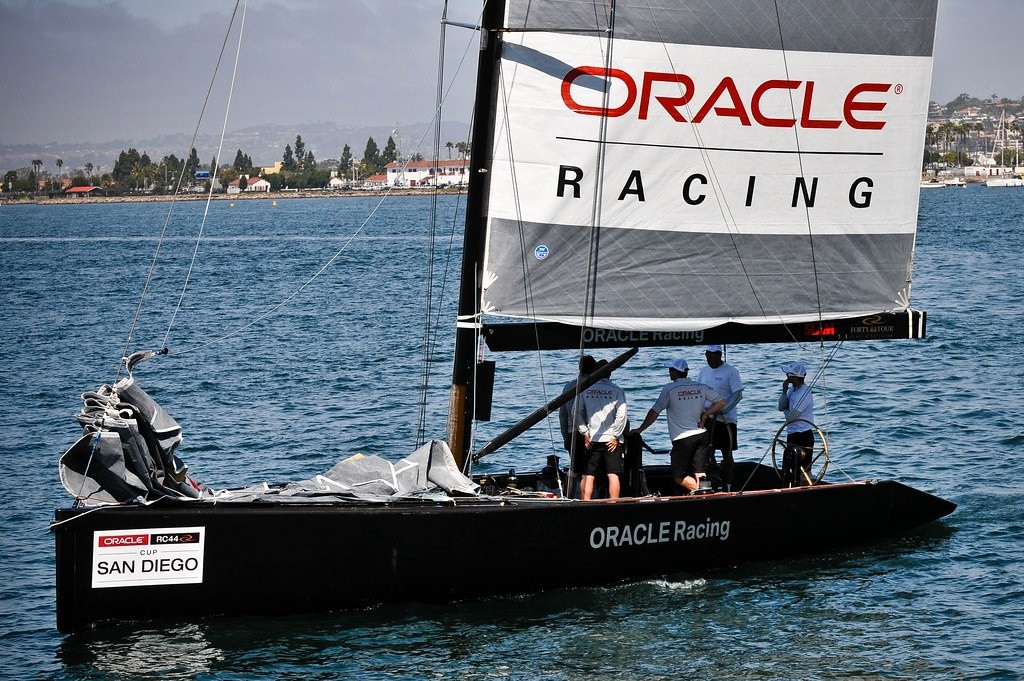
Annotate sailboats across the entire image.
[51,1,961,635]
[986,109,1024,186]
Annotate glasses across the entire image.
[785,373,795,377]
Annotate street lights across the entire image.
[393,130,405,188]
[162,161,167,182]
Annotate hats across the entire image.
[702,345,722,353]
[783,364,807,378]
[665,358,689,372]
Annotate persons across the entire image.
[559,355,627,501]
[631,359,726,493]
[698,345,744,492]
[778,364,815,489]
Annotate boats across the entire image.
[921,175,967,190]
